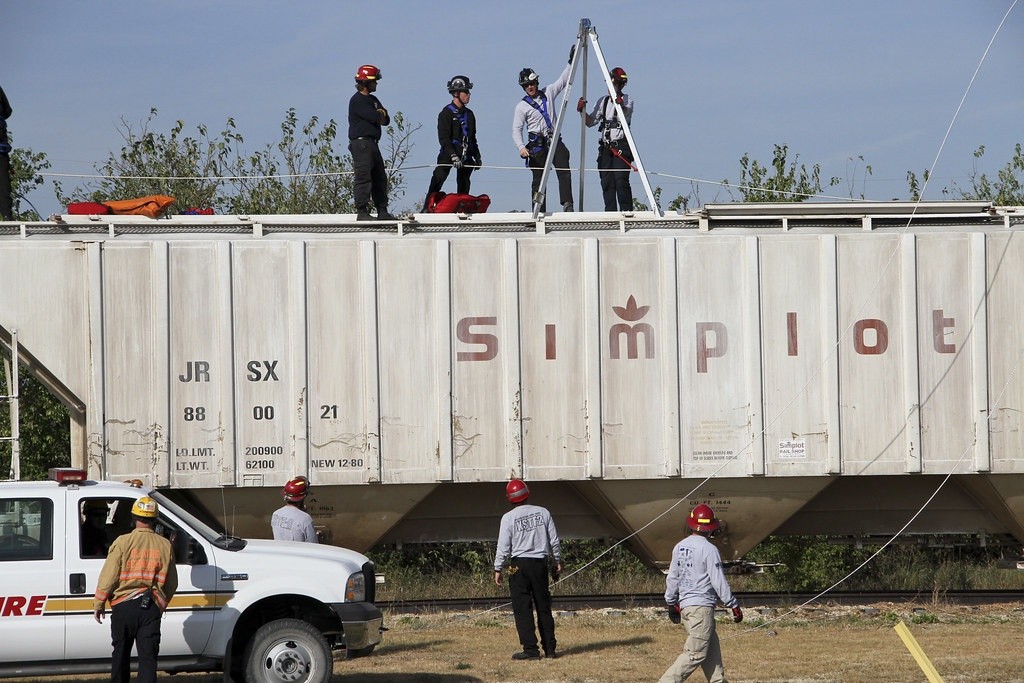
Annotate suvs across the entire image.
[0,468,390,683]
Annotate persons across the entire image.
[0,86,15,221]
[577,68,633,211]
[93,497,178,683]
[658,504,743,683]
[82,499,108,557]
[271,476,318,543]
[512,44,576,212]
[494,480,562,659]
[420,76,482,212]
[348,64,397,220]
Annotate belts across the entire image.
[355,135,379,144]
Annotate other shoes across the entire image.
[545,648,557,659]
[3,215,16,221]
[563,202,574,213]
[511,650,540,661]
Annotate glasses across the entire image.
[523,81,536,87]
[91,512,106,517]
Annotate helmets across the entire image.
[82,499,110,515]
[281,476,310,501]
[608,68,628,90]
[354,64,382,81]
[130,497,159,518]
[506,479,530,503]
[518,68,539,89]
[686,503,720,532]
[447,76,474,92]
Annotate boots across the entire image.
[357,207,378,221]
[377,206,394,220]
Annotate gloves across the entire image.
[577,97,588,113]
[472,153,481,170]
[608,92,624,105]
[450,152,464,170]
[668,605,681,624]
[731,605,743,624]
[567,44,575,65]
